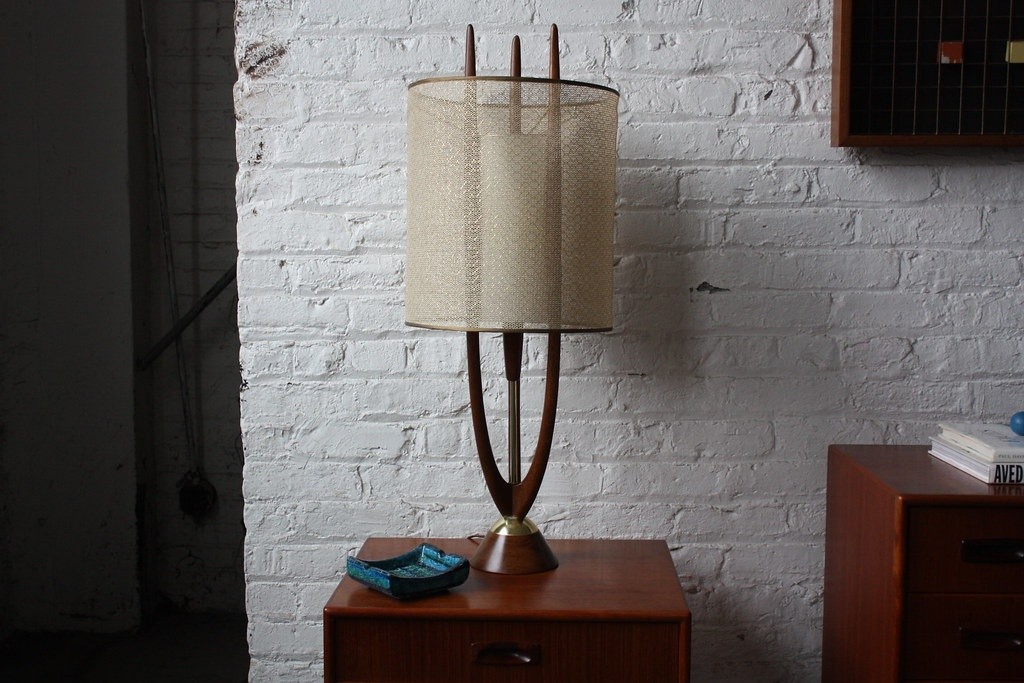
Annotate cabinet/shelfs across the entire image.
[321,538,692,683]
[820,442,1024,683]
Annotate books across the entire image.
[928,421,1024,484]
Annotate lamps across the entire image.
[402,21,621,572]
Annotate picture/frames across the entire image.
[830,0,1024,148]
